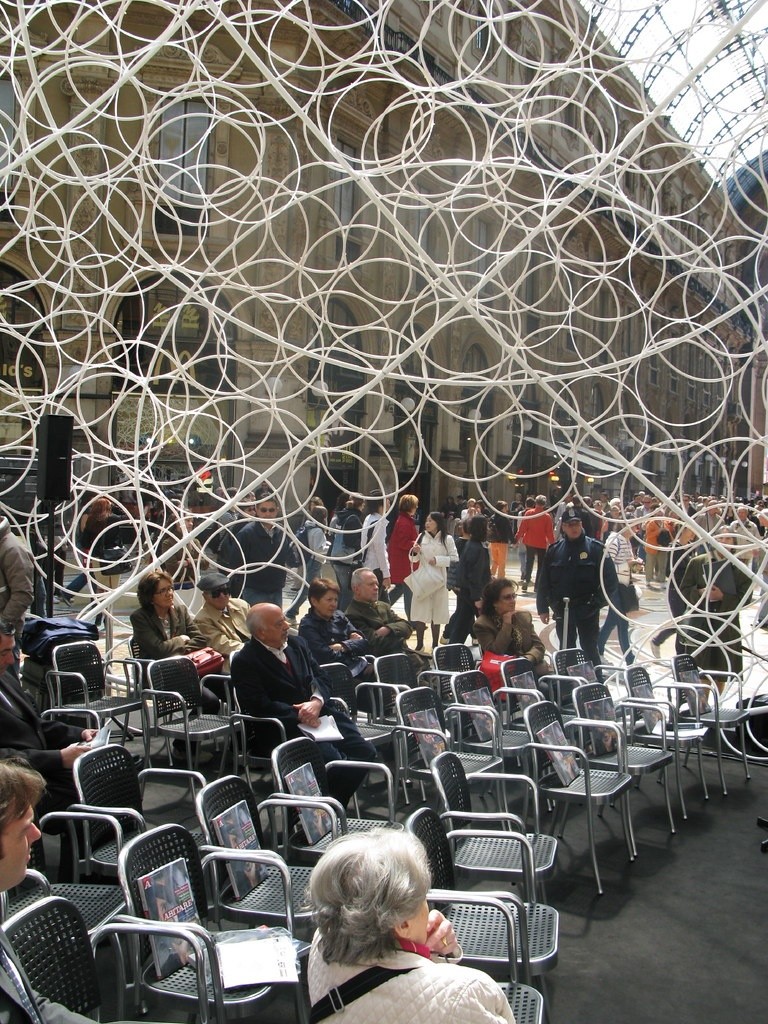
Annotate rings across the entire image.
[441,936,449,947]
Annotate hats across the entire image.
[561,508,581,522]
[197,573,229,591]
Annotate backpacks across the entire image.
[296,525,319,564]
[655,521,672,547]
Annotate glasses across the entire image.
[259,507,278,512]
[211,587,229,598]
[155,586,174,597]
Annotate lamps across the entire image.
[389,398,415,418]
[615,437,635,453]
[304,380,329,411]
[554,427,573,440]
[265,376,284,394]
[507,419,533,436]
[687,451,748,468]
[453,409,481,422]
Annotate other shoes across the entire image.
[599,655,614,666]
[440,637,448,644]
[522,581,527,590]
[650,639,660,659]
[415,644,425,652]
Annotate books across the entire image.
[566,660,600,688]
[138,858,300,989]
[632,683,662,734]
[212,800,270,898]
[511,669,540,709]
[407,707,451,770]
[297,715,344,742]
[584,695,624,756]
[678,669,713,715]
[67,718,112,749]
[283,761,338,844]
[535,719,580,786]
[462,686,503,743]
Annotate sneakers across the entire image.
[60,588,72,606]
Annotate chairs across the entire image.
[0,637,751,1024]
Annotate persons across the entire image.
[145,664,708,980]
[129,570,231,762]
[306,820,515,1024]
[30,486,768,717]
[0,755,106,1024]
[0,621,140,884]
[231,602,379,843]
[0,516,35,690]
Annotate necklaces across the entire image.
[157,615,169,626]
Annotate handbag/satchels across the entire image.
[186,647,225,677]
[619,581,640,613]
[479,650,516,702]
[100,548,133,576]
[331,514,363,565]
[403,546,444,602]
